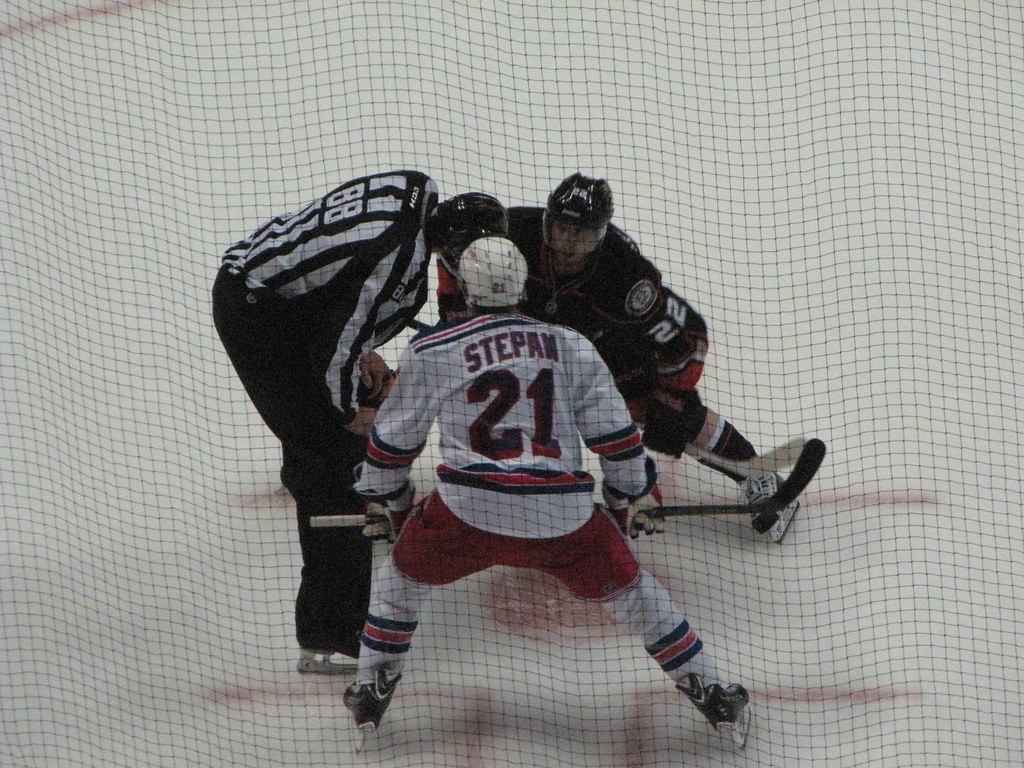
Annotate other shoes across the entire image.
[296,648,359,674]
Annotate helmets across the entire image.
[547,173,615,228]
[459,237,528,307]
[437,193,509,247]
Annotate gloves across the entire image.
[602,457,666,539]
[352,461,416,542]
[642,388,702,458]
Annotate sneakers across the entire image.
[676,672,751,749]
[343,666,402,754]
[738,470,799,545]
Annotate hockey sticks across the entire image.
[403,316,809,482]
[308,434,829,533]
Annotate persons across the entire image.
[212,170,509,676]
[436,172,800,543]
[344,237,751,756]
